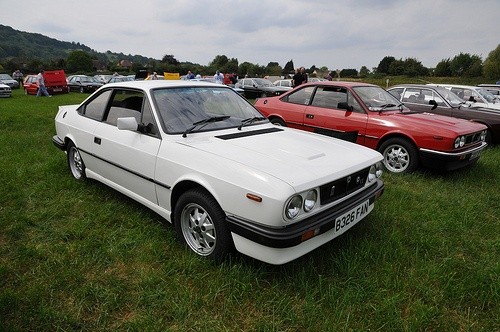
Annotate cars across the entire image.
[23,70,67,96]
[234,78,292,100]
[424,84,499,112]
[180,75,224,84]
[66,75,104,94]
[253,80,487,176]
[95,75,164,84]
[0,74,19,89]
[0,81,13,97]
[380,84,500,148]
[51,81,387,265]
[270,80,299,87]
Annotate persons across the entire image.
[186,71,195,81]
[36,69,52,98]
[292,67,308,88]
[196,72,207,80]
[327,72,337,81]
[213,70,239,86]
[150,72,158,80]
[13,70,25,89]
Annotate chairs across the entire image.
[323,87,337,98]
[408,94,425,103]
[453,91,464,99]
[119,96,144,113]
